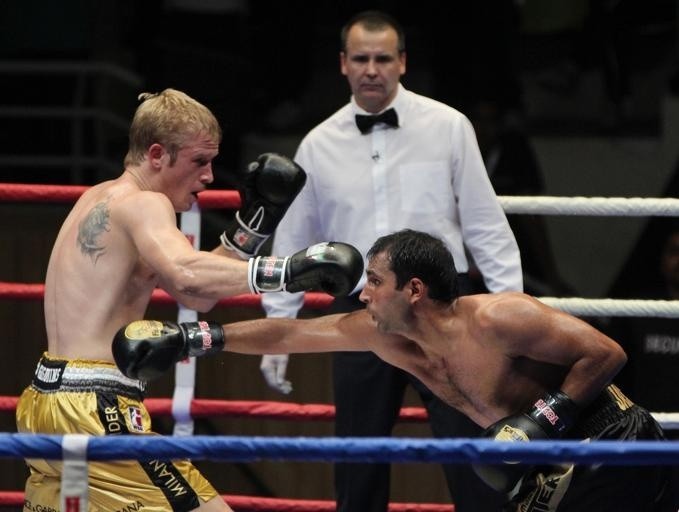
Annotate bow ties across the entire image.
[354,107,403,135]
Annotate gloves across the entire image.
[246,239,363,301]
[110,317,226,384]
[458,388,585,497]
[213,150,306,264]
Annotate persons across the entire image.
[444,79,554,298]
[618,231,679,441]
[16,86,364,510]
[111,230,679,509]
[258,17,523,510]
